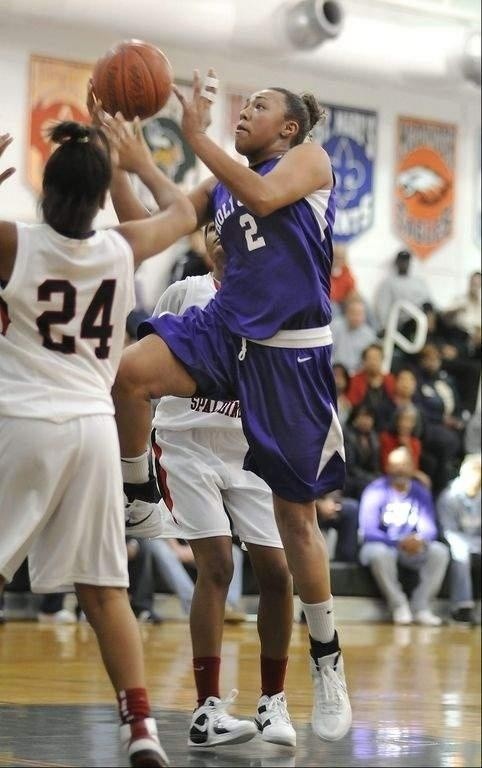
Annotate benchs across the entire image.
[0,555,482,626]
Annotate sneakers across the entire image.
[308,650,352,742]
[414,604,442,625]
[253,692,297,747]
[118,718,169,766]
[187,688,257,749]
[392,603,413,624]
[123,476,166,541]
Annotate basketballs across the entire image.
[92,38,175,120]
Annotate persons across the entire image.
[86,68,353,742]
[0,110,197,767]
[317,250,482,626]
[150,218,299,747]
[38,537,249,626]
[171,228,212,281]
